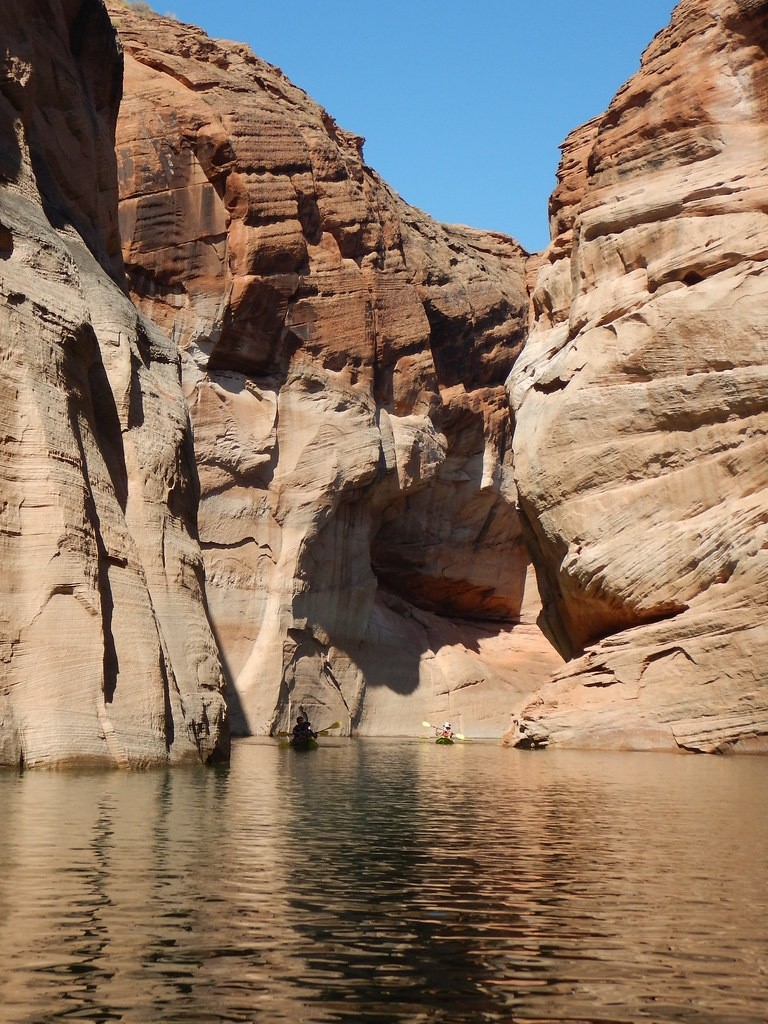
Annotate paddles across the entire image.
[419,735,456,740]
[278,721,342,750]
[279,730,329,738]
[422,721,465,740]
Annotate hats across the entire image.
[443,722,452,731]
[302,722,311,729]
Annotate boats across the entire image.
[289,737,319,751]
[435,736,455,745]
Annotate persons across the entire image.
[293,717,317,739]
[436,723,453,739]
